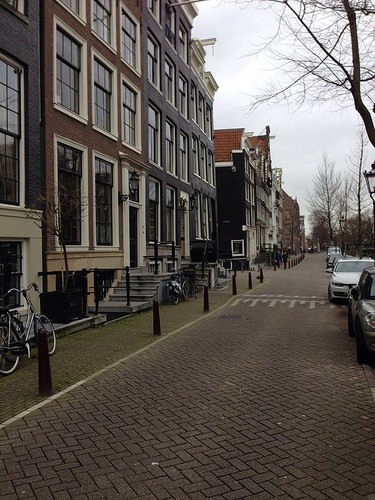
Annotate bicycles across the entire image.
[0,282,57,375]
[166,265,199,305]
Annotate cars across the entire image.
[325,246,375,302]
[347,267,375,363]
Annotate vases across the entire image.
[38,289,84,325]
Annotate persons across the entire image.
[275,250,282,268]
[283,248,289,262]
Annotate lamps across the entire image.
[118,169,139,205]
[189,193,198,211]
